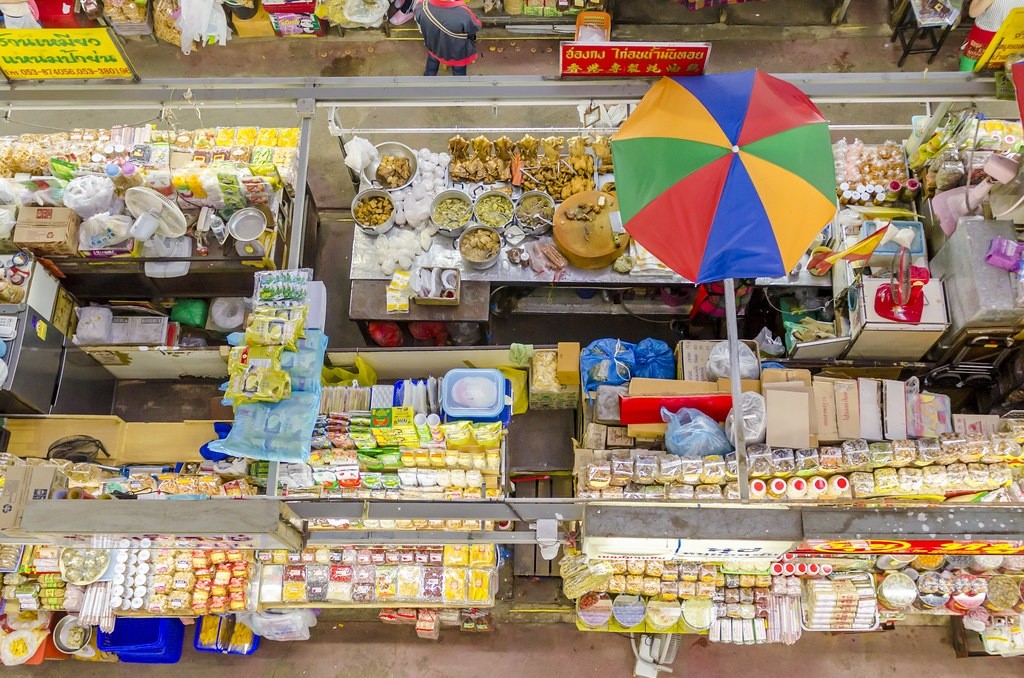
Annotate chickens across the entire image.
[448,134,613,165]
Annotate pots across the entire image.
[351,180,396,235]
[514,183,556,235]
[452,225,508,270]
[474,185,515,233]
[429,182,474,237]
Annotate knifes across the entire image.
[609,211,626,247]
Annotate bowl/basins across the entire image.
[505,226,525,245]
[363,141,419,192]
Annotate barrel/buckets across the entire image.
[876,555,1024,613]
[808,246,833,276]
[577,592,718,632]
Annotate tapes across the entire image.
[66,487,84,500]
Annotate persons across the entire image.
[958,0,1024,72]
[413,0,482,77]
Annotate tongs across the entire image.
[558,158,577,177]
[520,167,546,183]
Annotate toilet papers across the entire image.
[211,297,244,329]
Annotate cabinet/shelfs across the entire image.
[789,277,951,361]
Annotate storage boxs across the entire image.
[528,339,1005,451]
[34,0,154,36]
[393,367,513,427]
[96,616,262,663]
[253,280,327,333]
[231,0,327,37]
[0,204,139,265]
[856,220,927,266]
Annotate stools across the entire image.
[890,0,956,67]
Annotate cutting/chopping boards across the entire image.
[553,190,630,269]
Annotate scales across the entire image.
[228,207,272,257]
[53,615,101,661]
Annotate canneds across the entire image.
[521,253,530,267]
[837,183,886,206]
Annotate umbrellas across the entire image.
[610,68,840,286]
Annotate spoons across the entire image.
[506,233,530,238]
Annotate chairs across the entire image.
[575,11,611,42]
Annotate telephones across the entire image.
[197,207,214,231]
[0,316,17,341]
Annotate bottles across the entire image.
[992,135,1024,155]
[499,521,514,532]
[770,554,832,576]
[503,551,512,559]
[836,181,918,206]
[806,233,826,255]
[521,252,529,267]
[92,144,141,192]
[747,475,849,501]
[820,227,827,246]
[210,214,227,245]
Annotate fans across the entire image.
[932,146,1024,239]
[47,434,105,465]
[125,186,193,278]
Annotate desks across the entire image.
[0,413,235,468]
[0,611,72,666]
[350,151,832,345]
